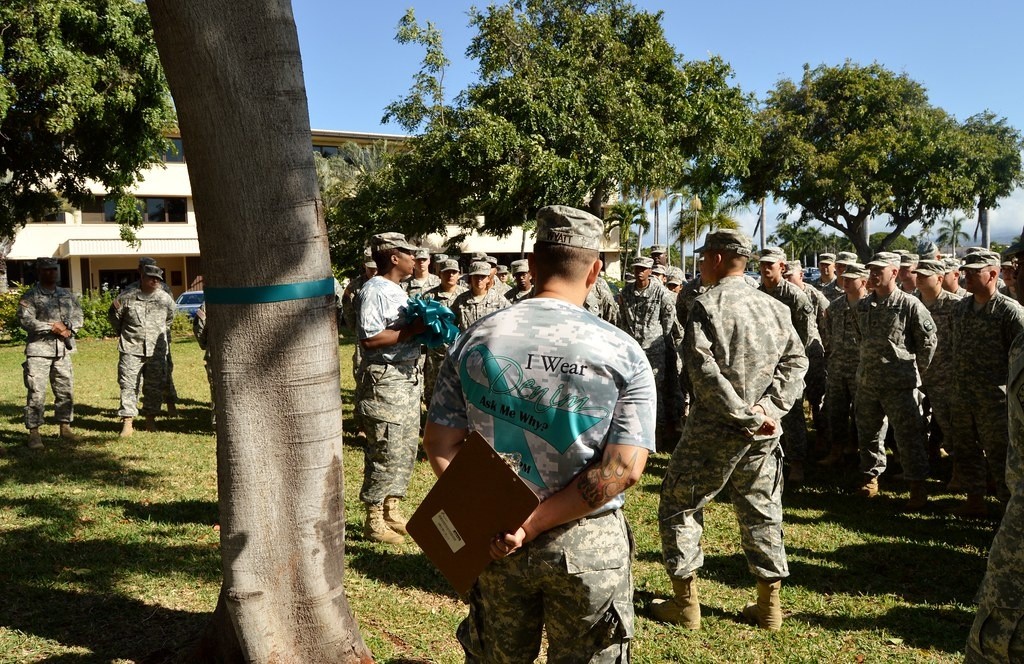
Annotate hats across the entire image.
[757,245,1021,277]
[693,229,754,261]
[36,257,59,269]
[535,205,605,250]
[361,231,529,276]
[630,244,682,286]
[138,256,163,279]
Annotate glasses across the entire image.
[961,270,982,275]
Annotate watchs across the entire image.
[63,321,69,329]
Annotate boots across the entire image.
[383,497,411,535]
[859,476,879,498]
[25,428,44,449]
[906,481,927,509]
[654,424,663,450]
[785,434,853,484]
[364,502,403,544]
[946,463,964,490]
[744,579,782,631]
[648,577,702,629]
[120,417,134,438]
[965,492,985,516]
[168,402,177,415]
[147,416,158,433]
[60,422,79,440]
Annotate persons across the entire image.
[333,246,536,410]
[353,232,427,544]
[584,241,1024,518]
[424,205,655,664]
[192,301,217,430]
[650,228,809,633]
[963,227,1024,664]
[108,257,178,438]
[17,258,84,450]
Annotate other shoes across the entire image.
[890,471,907,482]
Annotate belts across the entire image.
[369,360,419,367]
